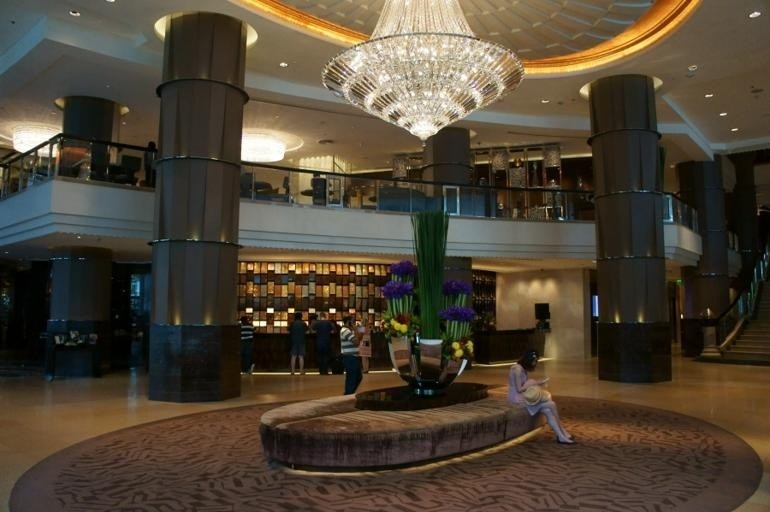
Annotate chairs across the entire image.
[106,155,141,185]
[54,138,92,182]
[240,172,272,197]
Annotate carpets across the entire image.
[9,393,765,512]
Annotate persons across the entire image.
[338,313,366,396]
[143,140,161,184]
[286,312,309,376]
[356,313,373,374]
[310,315,335,376]
[506,347,578,443]
[330,317,346,375]
[238,316,258,375]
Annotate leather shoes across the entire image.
[556,435,578,445]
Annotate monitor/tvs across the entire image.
[534,302,552,321]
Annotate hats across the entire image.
[523,384,543,406]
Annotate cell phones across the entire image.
[349,321,352,327]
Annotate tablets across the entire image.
[538,376,550,385]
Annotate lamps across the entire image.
[493,152,506,174]
[322,1,524,149]
[545,143,560,168]
[392,153,407,181]
[509,151,526,189]
[11,120,61,159]
[241,128,304,163]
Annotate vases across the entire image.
[387,337,469,397]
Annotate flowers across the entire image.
[440,280,476,366]
[378,260,423,344]
